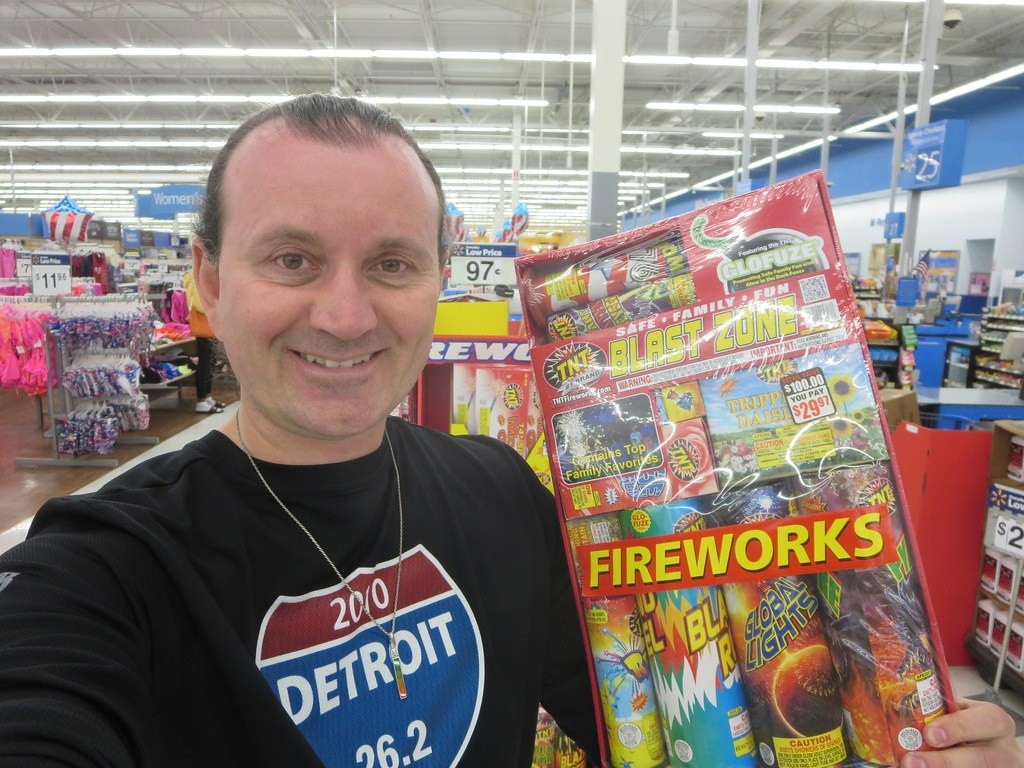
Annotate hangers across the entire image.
[67,387,149,420]
[0,238,186,313]
[72,347,139,373]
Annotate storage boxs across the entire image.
[988,420,1024,491]
[452,168,968,768]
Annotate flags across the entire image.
[914,251,929,304]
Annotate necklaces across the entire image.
[236,408,408,701]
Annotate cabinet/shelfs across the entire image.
[858,317,903,390]
[972,298,1024,389]
[137,336,196,409]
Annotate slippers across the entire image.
[214,401,226,407]
[197,406,224,414]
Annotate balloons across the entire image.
[444,202,530,243]
[42,195,93,244]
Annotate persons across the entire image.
[0,94,1023,767]
[182,271,226,414]
